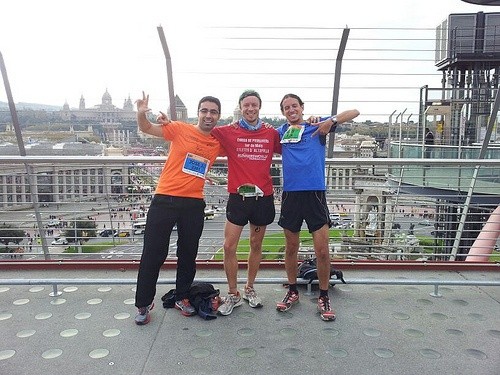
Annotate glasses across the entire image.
[197,109,219,115]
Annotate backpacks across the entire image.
[297,258,347,291]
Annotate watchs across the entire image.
[331,115,338,125]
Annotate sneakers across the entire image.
[217,291,243,316]
[242,285,263,308]
[173,298,198,316]
[276,289,300,312]
[316,295,336,321]
[134,302,154,325]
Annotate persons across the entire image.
[155,91,325,317]
[230,94,359,321]
[135,91,274,326]
